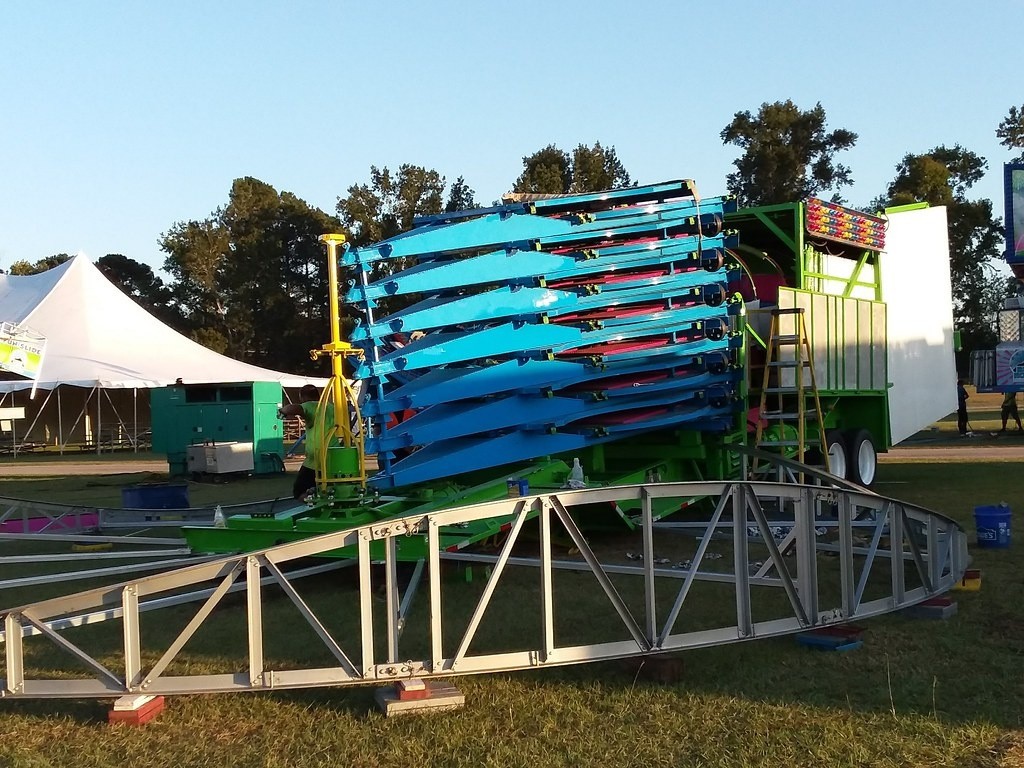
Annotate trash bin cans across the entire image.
[120,483,190,509]
[973,505,1012,549]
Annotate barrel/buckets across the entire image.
[973,504,1012,549]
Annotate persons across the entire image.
[382,408,422,463]
[956,380,972,438]
[278,384,335,503]
[1000,391,1022,432]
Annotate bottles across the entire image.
[214,504,228,527]
[572,458,583,482]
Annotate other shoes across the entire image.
[960,431,968,436]
[999,429,1007,433]
[1018,427,1023,431]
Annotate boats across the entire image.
[338,182,746,492]
